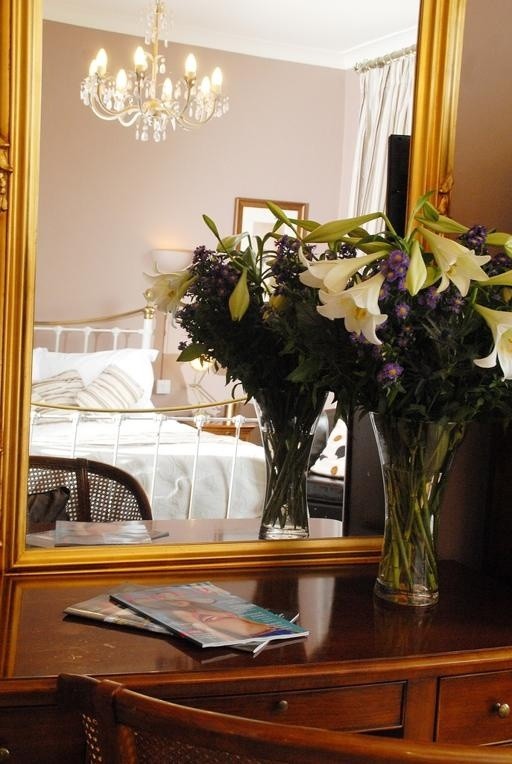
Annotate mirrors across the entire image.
[0,0,467,578]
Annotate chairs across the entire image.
[24,454,153,521]
[57,669,511,763]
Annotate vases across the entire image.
[370,411,466,608]
[254,392,328,542]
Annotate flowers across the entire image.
[143,217,328,390]
[278,194,512,422]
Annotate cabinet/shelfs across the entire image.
[0,561,512,764]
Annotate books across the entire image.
[25,526,170,546]
[110,581,311,649]
[55,519,152,545]
[62,583,299,654]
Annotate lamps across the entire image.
[80,0,228,143]
